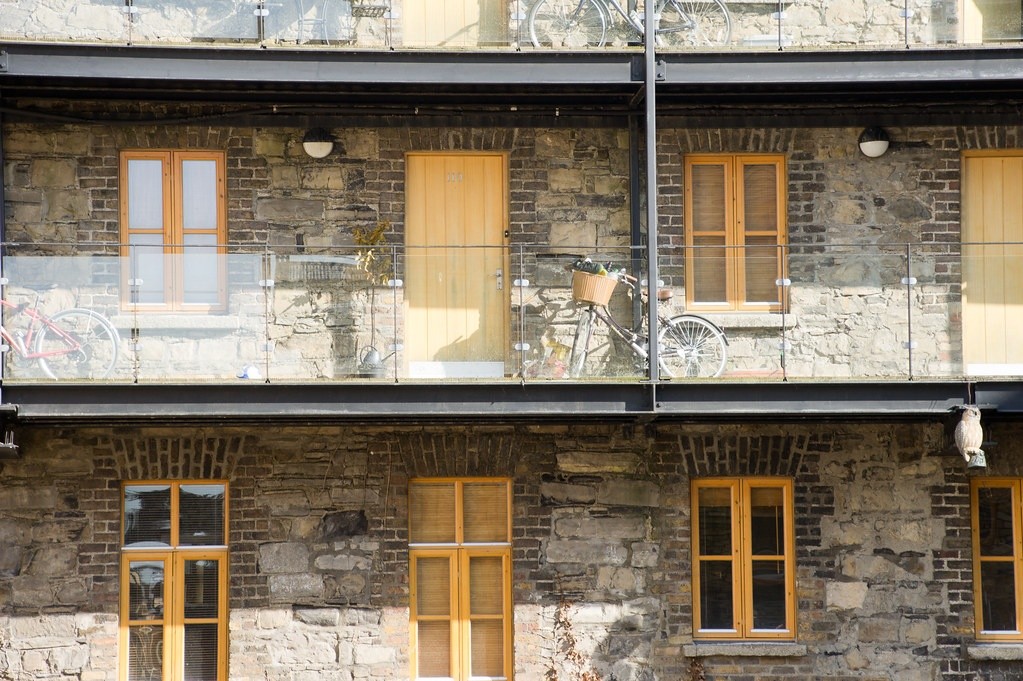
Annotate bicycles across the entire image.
[564,259,731,377]
[1,277,122,382]
[529,0,732,49]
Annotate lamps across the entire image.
[303,128,335,159]
[858,125,890,158]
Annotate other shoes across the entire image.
[573,259,603,274]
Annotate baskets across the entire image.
[574,271,618,306]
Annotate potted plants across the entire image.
[350,218,393,378]
